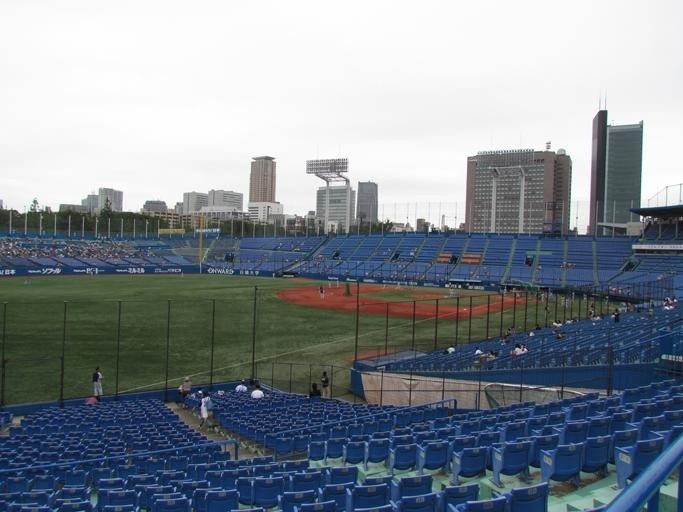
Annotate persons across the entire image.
[318,286,324,299]
[235,382,247,392]
[442,287,679,364]
[308,382,321,398]
[181,374,191,410]
[0,235,154,257]
[266,239,576,289]
[92,366,104,395]
[250,385,264,399]
[320,371,331,398]
[196,390,212,431]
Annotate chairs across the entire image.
[0,232,683,512]
